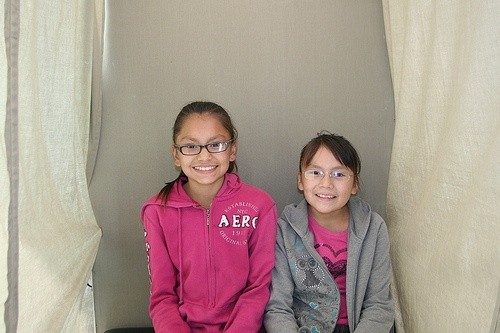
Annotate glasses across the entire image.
[173,139,234,157]
[302,169,355,181]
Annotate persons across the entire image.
[262,130,395,332]
[141,102,277,333]
